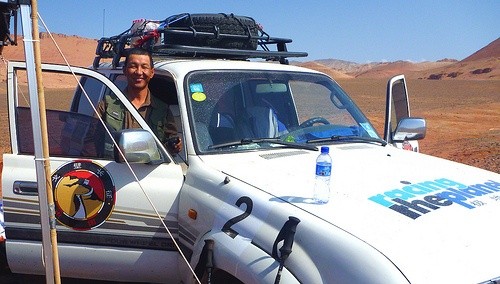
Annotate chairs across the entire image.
[171,105,214,163]
[230,90,288,141]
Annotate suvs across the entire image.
[2,9,500,284]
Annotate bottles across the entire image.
[313,147,332,204]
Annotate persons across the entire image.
[80,48,183,166]
